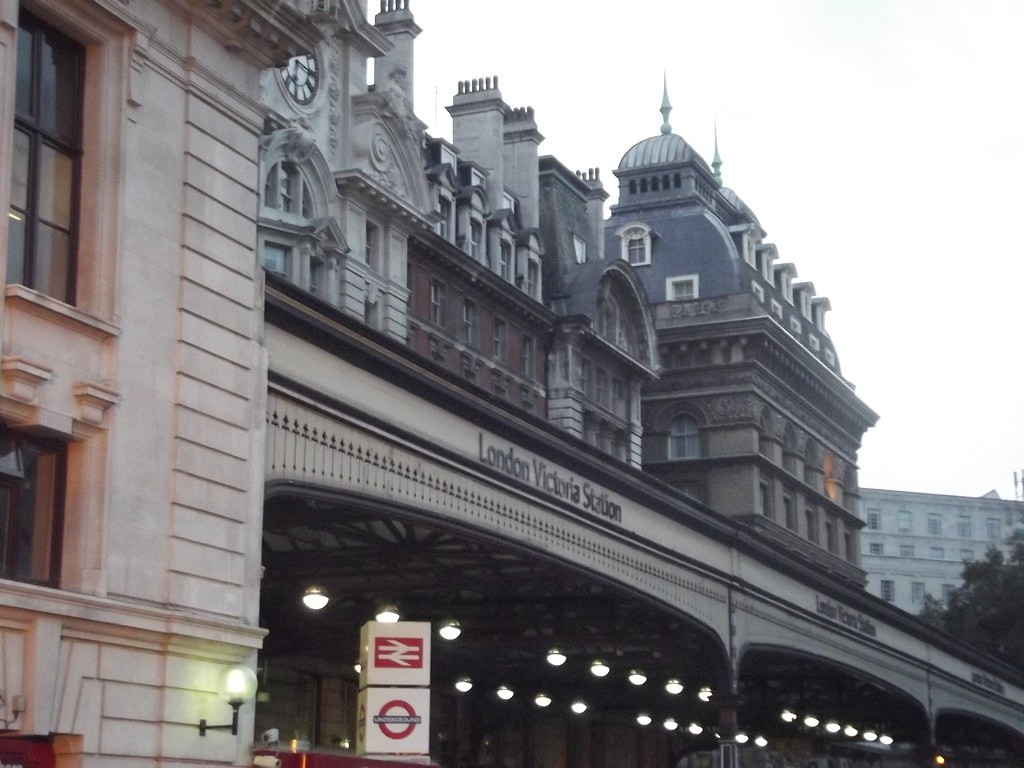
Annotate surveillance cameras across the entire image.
[262,728,279,744]
[253,756,281,768]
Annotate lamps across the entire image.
[200,663,258,736]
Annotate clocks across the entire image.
[279,50,320,105]
